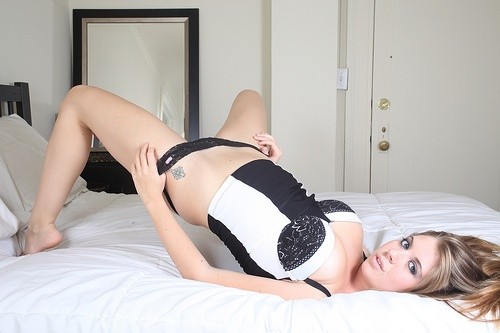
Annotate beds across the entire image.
[0,81,500,333]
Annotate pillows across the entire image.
[0,113,88,229]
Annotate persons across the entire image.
[23,85,500,322]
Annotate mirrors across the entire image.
[81,17,189,154]
